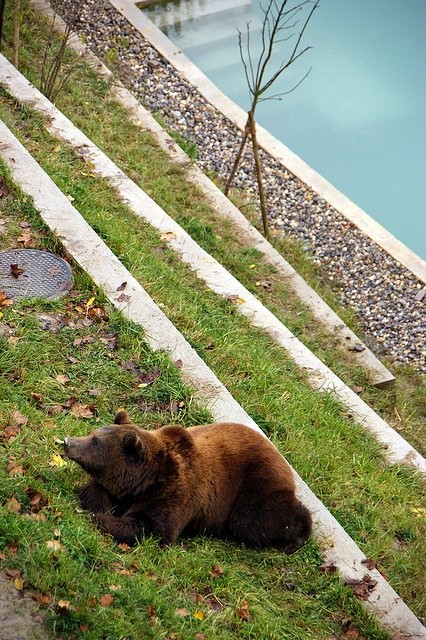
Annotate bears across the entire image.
[61,407,314,557]
